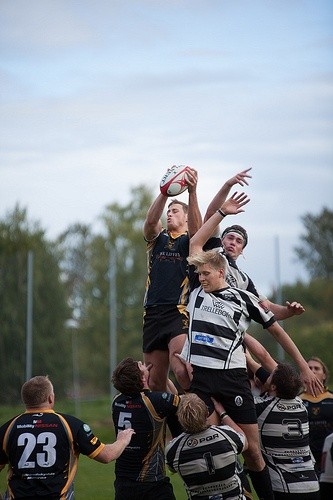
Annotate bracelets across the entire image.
[216,209,226,219]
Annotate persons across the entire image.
[165,392,251,500]
[219,412,229,420]
[0,375,136,500]
[204,168,304,322]
[243,349,278,402]
[181,191,324,500]
[143,166,201,392]
[237,331,323,500]
[296,357,333,500]
[320,433,333,500]
[112,352,194,500]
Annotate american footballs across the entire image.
[160,164,190,196]
